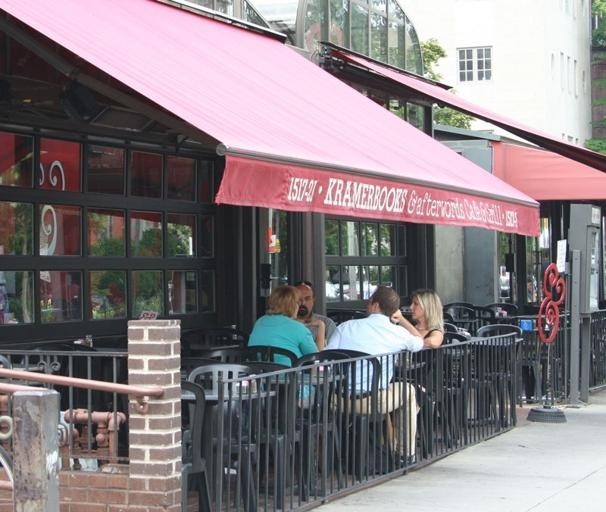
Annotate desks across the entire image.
[468,336,526,352]
[396,360,427,373]
[181,383,278,511]
[267,372,346,389]
[185,343,239,358]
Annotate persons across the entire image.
[391,287,445,349]
[247,286,326,409]
[304,286,421,464]
[527,278,537,302]
[295,280,336,343]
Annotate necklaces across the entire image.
[413,325,428,332]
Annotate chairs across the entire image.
[445,322,459,332]
[182,328,247,346]
[444,305,473,332]
[186,362,265,461]
[472,306,494,336]
[239,344,298,366]
[252,362,308,510]
[483,303,521,316]
[475,323,523,428]
[20,344,103,449]
[290,351,354,495]
[194,348,244,366]
[326,309,367,326]
[118,379,214,511]
[325,349,388,482]
[442,332,470,445]
